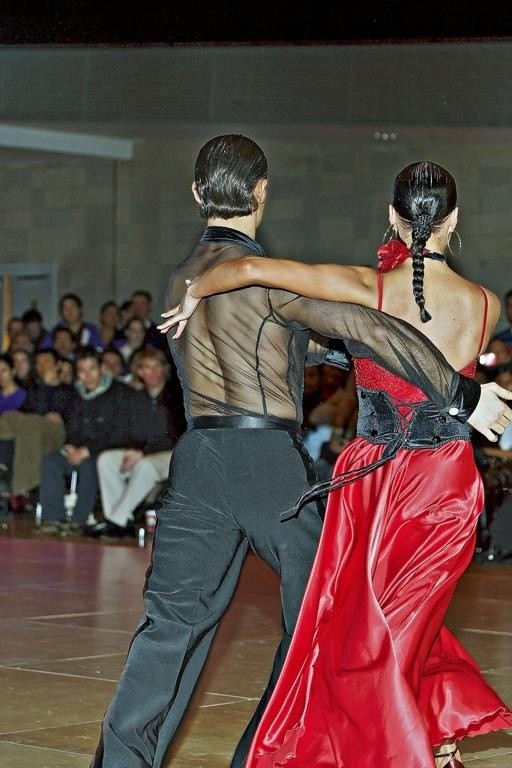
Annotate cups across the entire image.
[146,510,158,534]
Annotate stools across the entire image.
[30,471,82,532]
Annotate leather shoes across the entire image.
[434,746,466,768]
[30,518,138,537]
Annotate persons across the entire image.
[89,133,512,768]
[156,160,512,768]
